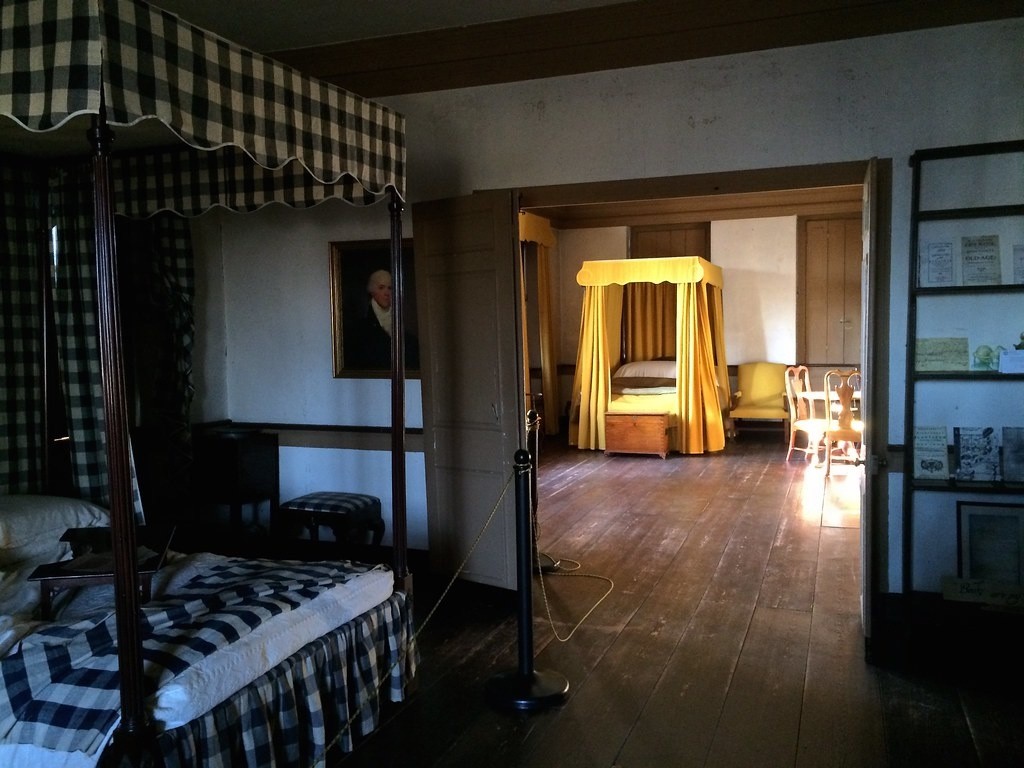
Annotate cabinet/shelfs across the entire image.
[903,140,1024,680]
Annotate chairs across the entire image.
[822,369,864,477]
[784,366,835,469]
[729,359,792,444]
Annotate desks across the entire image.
[28,525,177,622]
[798,389,861,468]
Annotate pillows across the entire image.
[0,491,112,569]
[612,360,678,387]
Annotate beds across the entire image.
[2,536,420,768]
[599,380,683,458]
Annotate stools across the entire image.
[267,489,386,565]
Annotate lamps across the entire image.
[192,428,278,555]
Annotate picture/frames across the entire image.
[955,497,1024,581]
[327,237,422,380]
